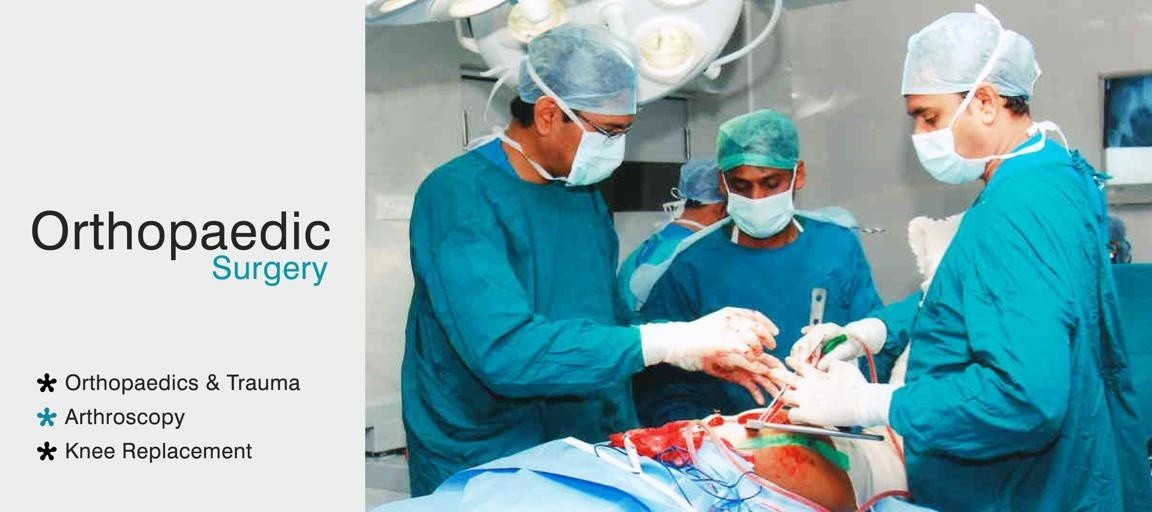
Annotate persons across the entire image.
[632,109,899,428]
[613,158,729,328]
[364,405,906,510]
[776,3,1152,511]
[401,21,788,498]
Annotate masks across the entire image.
[726,192,793,239]
[912,128,986,186]
[563,129,624,188]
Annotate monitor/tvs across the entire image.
[1095,67,1152,205]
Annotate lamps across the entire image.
[366,0,785,107]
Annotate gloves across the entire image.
[640,305,901,428]
